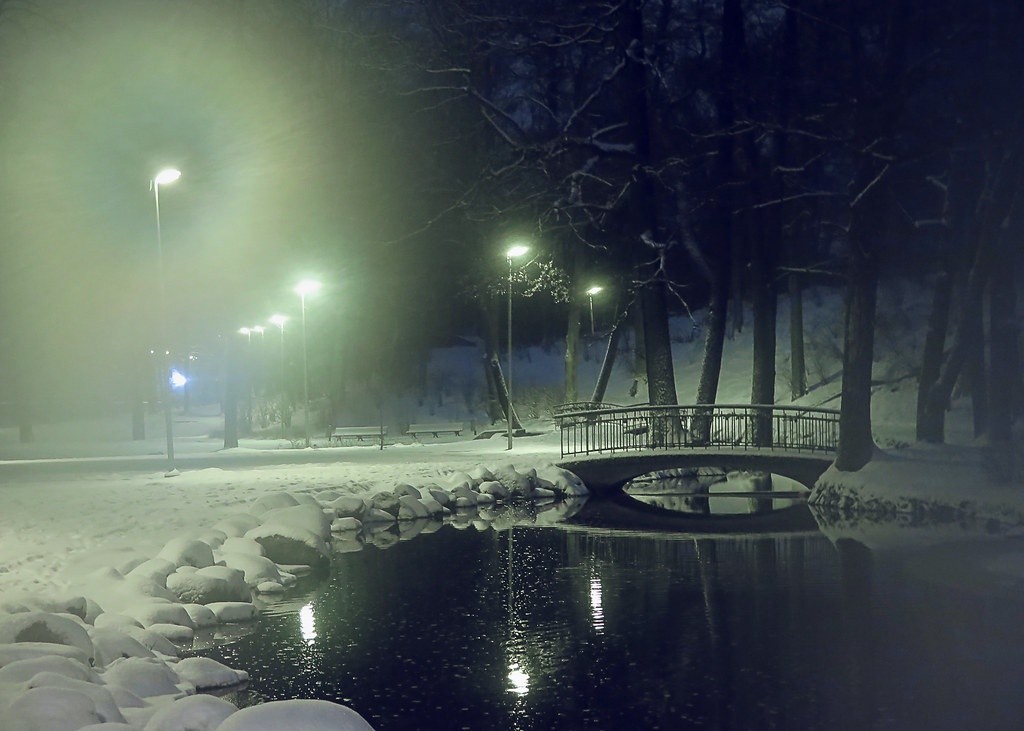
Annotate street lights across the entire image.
[271,314,283,440]
[240,326,263,429]
[298,281,321,448]
[586,287,603,335]
[506,246,529,449]
[154,167,180,476]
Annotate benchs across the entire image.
[332,426,387,441]
[406,424,464,438]
